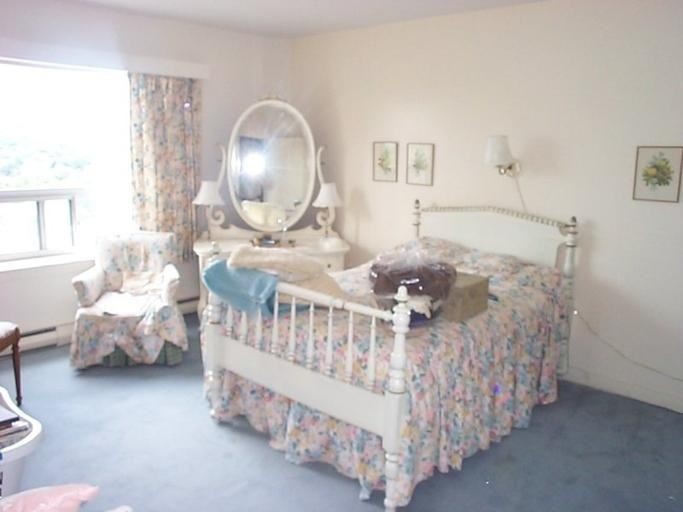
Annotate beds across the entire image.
[200,199,579,512]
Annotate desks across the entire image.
[193,227,352,321]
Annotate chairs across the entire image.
[0,321,23,408]
[69,230,189,370]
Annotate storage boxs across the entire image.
[431,273,490,324]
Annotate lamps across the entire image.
[312,183,344,226]
[483,133,522,178]
[192,180,226,226]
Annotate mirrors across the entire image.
[227,99,317,233]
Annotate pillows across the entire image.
[453,247,518,282]
[394,234,474,268]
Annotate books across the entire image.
[0,404,28,437]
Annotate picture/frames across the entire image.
[632,145,683,203]
[407,143,434,186]
[373,142,398,183]
